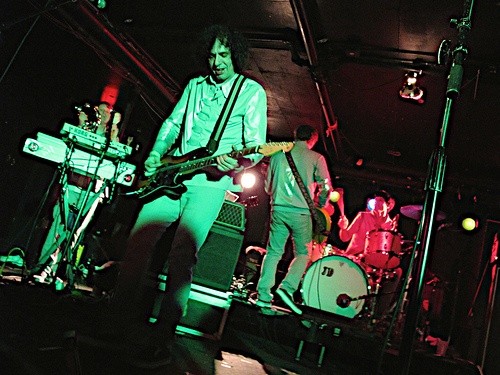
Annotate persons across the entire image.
[257,124,333,317]
[338,190,403,279]
[126,24,268,369]
[30,101,120,292]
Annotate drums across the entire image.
[365,229,401,268]
[302,253,370,320]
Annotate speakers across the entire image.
[192,224,244,290]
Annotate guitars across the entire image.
[256,164,332,246]
[121,142,294,197]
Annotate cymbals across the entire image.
[399,204,445,222]
[74,102,103,131]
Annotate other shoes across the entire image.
[127,346,173,368]
[78,325,133,352]
[256,299,273,308]
[276,287,302,316]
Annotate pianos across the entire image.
[61,122,134,158]
